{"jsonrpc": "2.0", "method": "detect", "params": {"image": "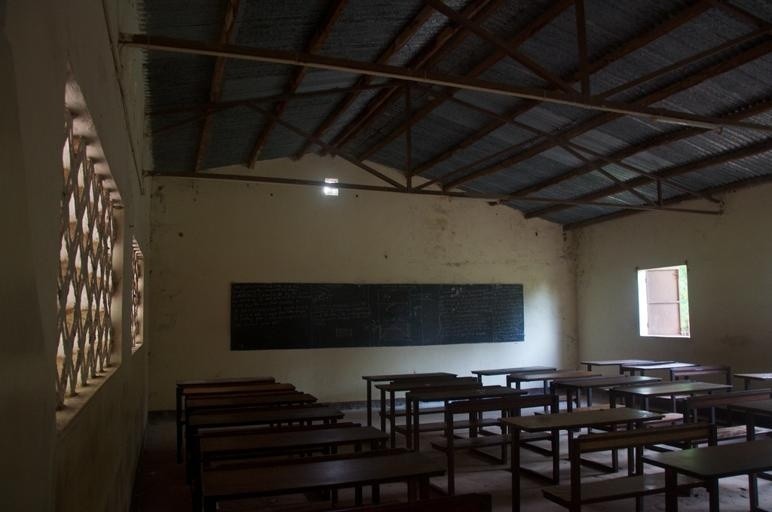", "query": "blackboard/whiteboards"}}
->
[230,283,524,350]
